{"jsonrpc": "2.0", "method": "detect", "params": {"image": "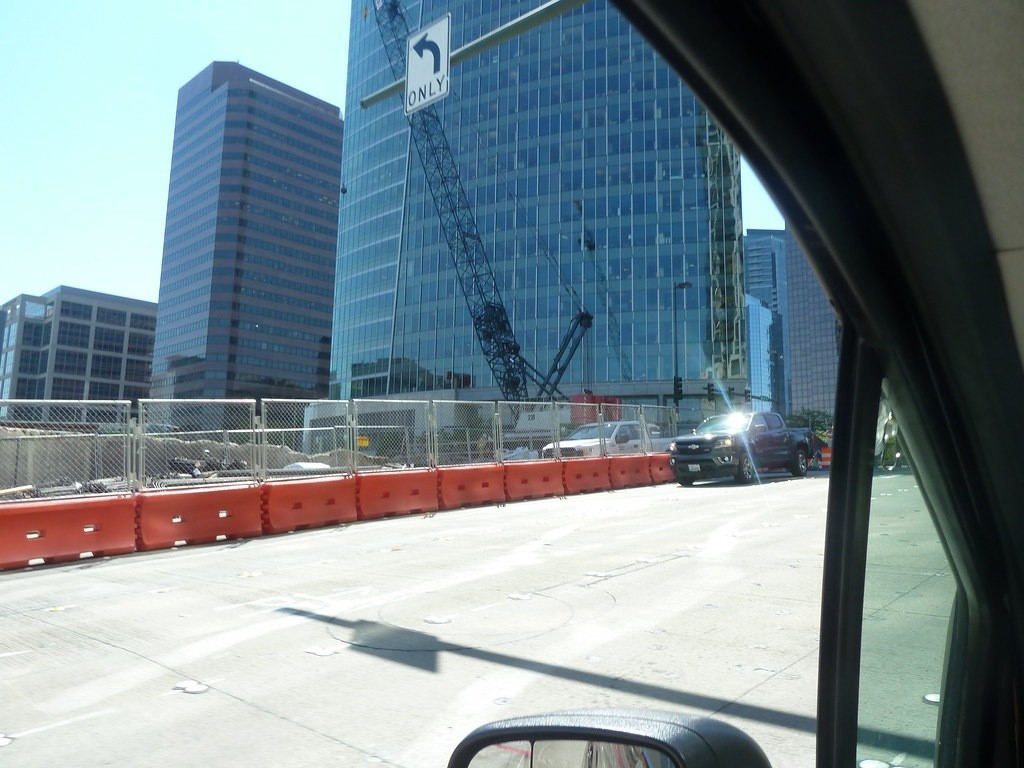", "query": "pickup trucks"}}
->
[542,420,672,459]
[669,412,814,486]
